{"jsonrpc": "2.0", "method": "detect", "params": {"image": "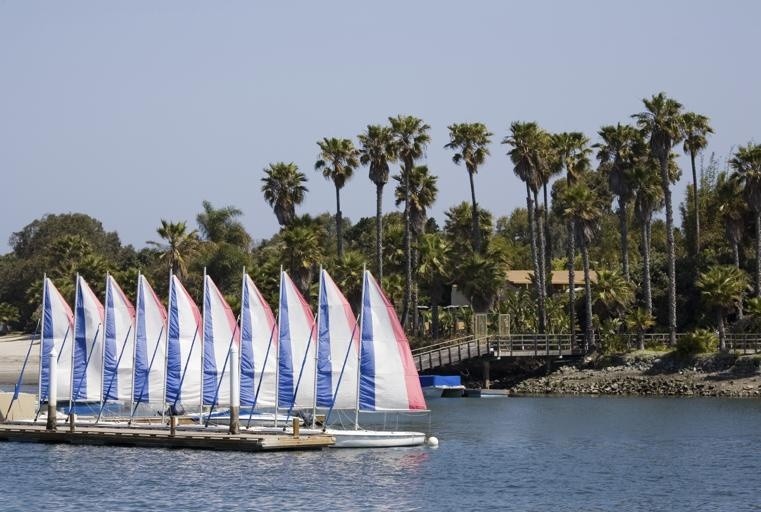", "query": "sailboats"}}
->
[275,260,432,448]
[0,264,362,447]
